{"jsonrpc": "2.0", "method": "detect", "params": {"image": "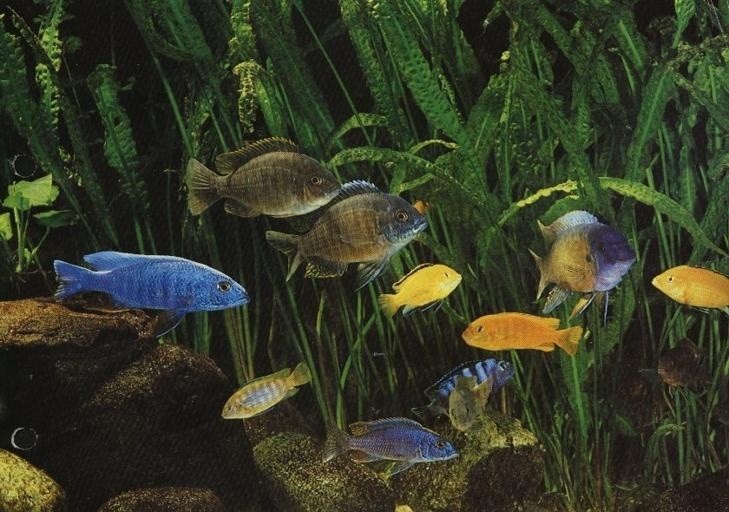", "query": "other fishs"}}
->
[184,136,342,218]
[651,264,729,316]
[321,416,460,478]
[657,336,709,400]
[527,209,637,330]
[53,250,251,338]
[462,311,584,357]
[266,180,461,316]
[410,356,517,432]
[221,362,313,420]
